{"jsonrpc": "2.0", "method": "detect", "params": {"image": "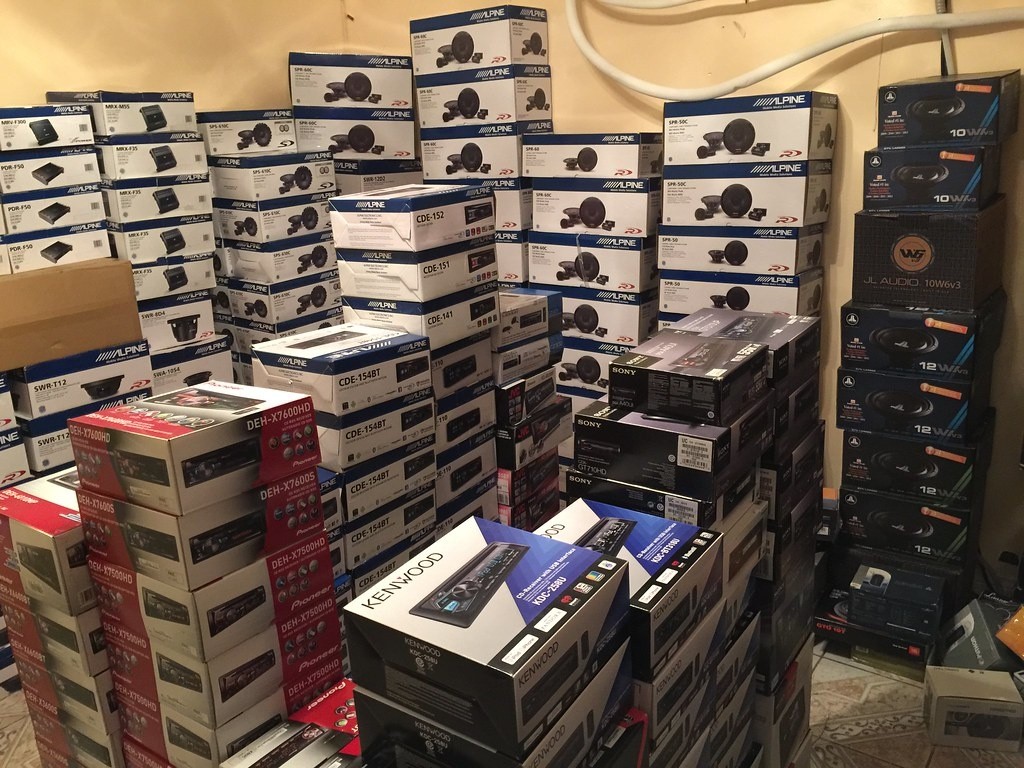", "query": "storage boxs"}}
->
[0,4,1024,768]
